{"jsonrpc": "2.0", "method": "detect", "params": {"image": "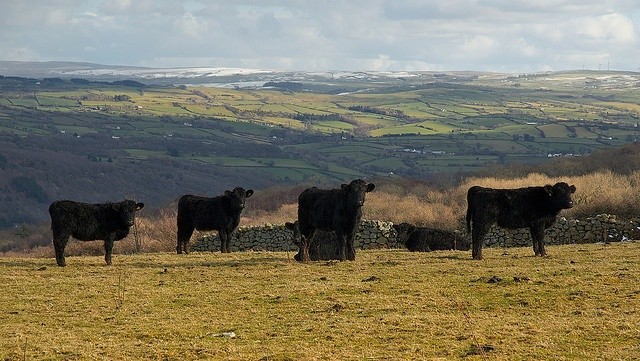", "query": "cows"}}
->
[466,182,576,259]
[297,179,375,265]
[177,187,254,255]
[48,199,145,267]
[391,223,470,252]
[285,220,356,261]
